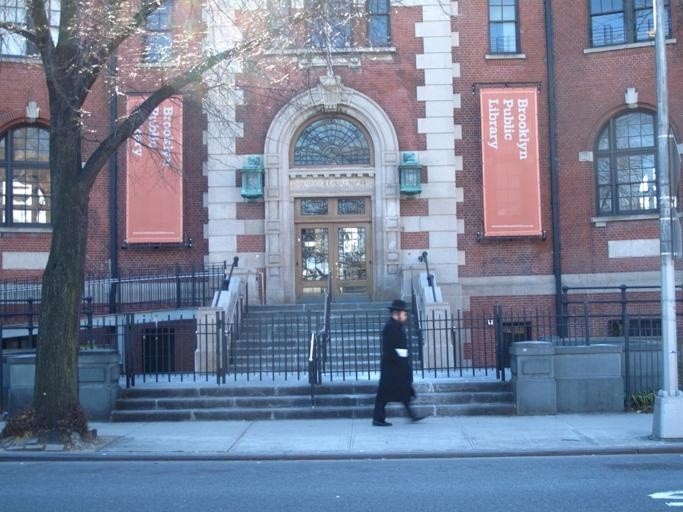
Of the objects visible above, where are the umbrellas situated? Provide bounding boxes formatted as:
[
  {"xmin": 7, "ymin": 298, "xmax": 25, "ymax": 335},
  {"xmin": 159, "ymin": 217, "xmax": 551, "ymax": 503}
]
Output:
[
  {"xmin": 413, "ymin": 415, "xmax": 424, "ymax": 420},
  {"xmin": 372, "ymin": 420, "xmax": 392, "ymax": 426}
]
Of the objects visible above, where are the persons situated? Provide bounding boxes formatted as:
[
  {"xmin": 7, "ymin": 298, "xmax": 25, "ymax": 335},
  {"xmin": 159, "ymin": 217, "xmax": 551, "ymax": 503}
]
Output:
[{"xmin": 372, "ymin": 299, "xmax": 433, "ymax": 426}]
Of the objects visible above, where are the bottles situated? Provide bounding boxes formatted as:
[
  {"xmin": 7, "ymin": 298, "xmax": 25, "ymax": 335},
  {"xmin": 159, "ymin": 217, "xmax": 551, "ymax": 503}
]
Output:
[{"xmin": 387, "ymin": 300, "xmax": 409, "ymax": 311}]
